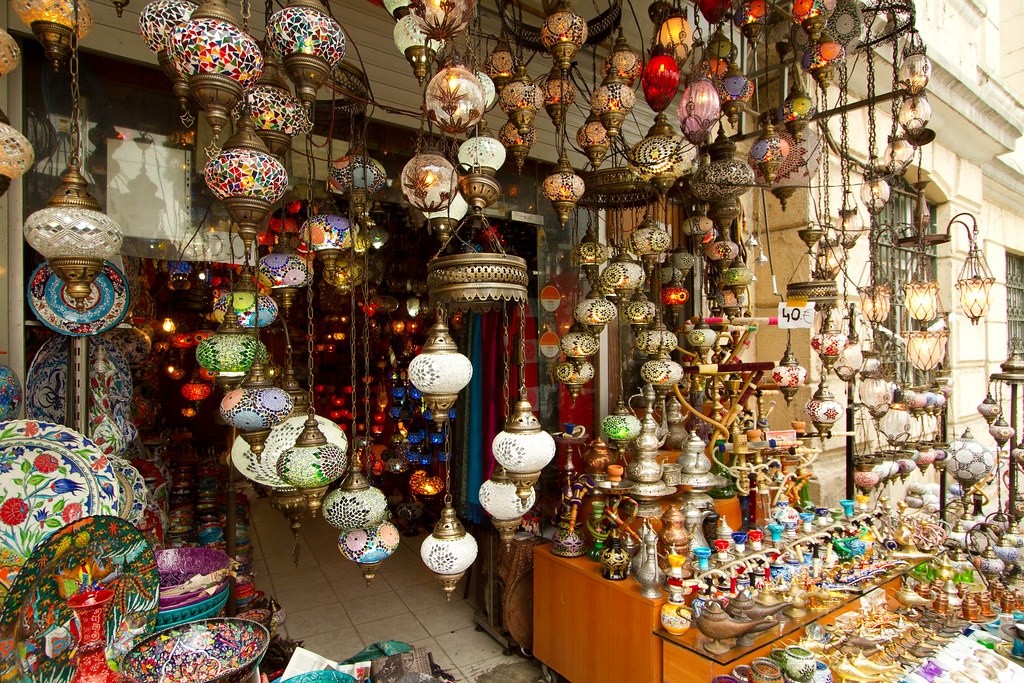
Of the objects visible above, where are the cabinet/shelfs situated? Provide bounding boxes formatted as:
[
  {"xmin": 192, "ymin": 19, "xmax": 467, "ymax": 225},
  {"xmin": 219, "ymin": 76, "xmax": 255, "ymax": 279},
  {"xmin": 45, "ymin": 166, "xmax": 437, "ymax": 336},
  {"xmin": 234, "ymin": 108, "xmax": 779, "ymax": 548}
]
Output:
[
  {"xmin": 652, "ymin": 544, "xmax": 972, "ymax": 683},
  {"xmin": 532, "ymin": 543, "xmax": 694, "ymax": 683}
]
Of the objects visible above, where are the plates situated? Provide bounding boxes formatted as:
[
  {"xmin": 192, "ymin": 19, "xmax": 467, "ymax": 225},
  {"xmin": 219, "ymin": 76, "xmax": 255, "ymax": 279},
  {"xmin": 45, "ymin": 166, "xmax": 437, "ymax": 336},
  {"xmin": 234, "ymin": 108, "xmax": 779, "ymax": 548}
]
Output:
[{"xmin": 0, "ymin": 261, "xmax": 266, "ymax": 683}]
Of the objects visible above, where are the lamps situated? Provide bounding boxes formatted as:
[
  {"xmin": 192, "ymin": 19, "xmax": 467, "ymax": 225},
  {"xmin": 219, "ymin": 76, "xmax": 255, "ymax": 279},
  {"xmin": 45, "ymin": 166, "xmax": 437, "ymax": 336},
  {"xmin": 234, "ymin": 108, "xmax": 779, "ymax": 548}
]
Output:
[{"xmin": 0, "ymin": 0, "xmax": 1024, "ymax": 604}]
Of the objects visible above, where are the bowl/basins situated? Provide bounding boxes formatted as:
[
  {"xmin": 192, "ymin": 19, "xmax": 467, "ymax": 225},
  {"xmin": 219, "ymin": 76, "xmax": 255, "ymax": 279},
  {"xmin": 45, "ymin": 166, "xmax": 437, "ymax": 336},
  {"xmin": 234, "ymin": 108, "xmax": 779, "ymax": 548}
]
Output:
[{"xmin": 169, "ymin": 466, "xmax": 271, "ymax": 627}]
